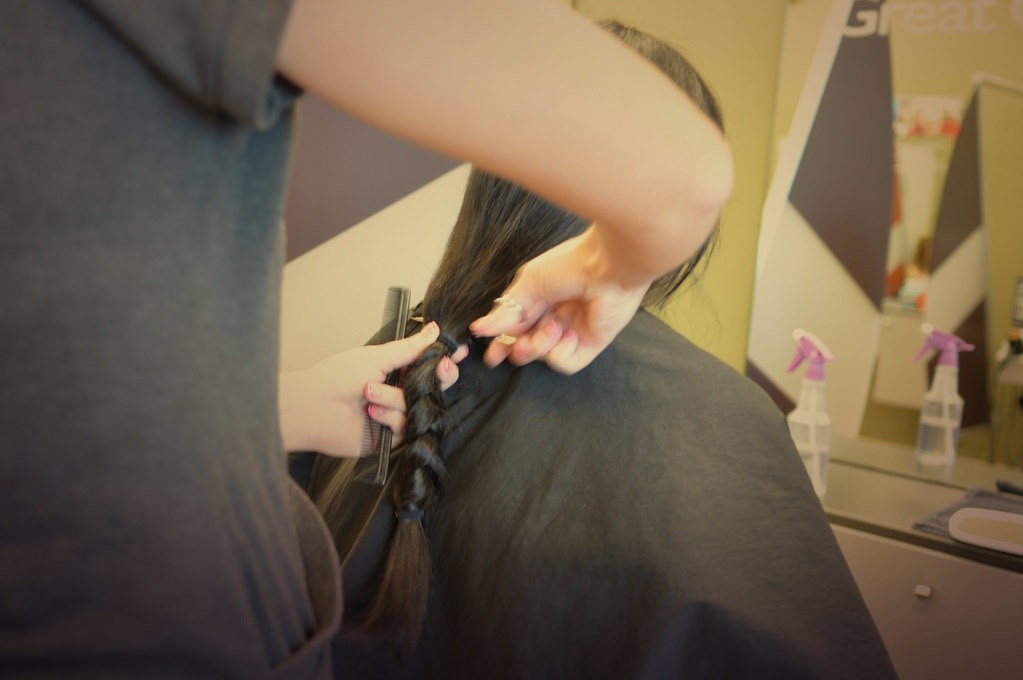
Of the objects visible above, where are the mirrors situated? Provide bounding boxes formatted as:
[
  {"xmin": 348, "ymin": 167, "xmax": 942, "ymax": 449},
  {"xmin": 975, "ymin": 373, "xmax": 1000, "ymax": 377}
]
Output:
[{"xmin": 741, "ymin": 0, "xmax": 1023, "ymax": 494}]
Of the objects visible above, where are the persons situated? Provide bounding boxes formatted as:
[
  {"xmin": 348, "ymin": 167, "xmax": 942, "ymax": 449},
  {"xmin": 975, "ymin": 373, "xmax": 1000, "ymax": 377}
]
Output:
[
  {"xmin": 1, "ymin": 0, "xmax": 735, "ymax": 679},
  {"xmin": 289, "ymin": 18, "xmax": 900, "ymax": 680}
]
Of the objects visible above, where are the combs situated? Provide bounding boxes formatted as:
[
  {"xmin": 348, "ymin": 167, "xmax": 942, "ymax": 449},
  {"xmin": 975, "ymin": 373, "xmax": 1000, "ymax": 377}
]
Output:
[{"xmin": 354, "ymin": 286, "xmax": 411, "ymax": 485}]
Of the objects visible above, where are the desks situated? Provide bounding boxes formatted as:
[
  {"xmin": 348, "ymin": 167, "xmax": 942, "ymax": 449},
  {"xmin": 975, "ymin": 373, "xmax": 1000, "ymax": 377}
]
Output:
[{"xmin": 821, "ymin": 459, "xmax": 1023, "ymax": 680}]
